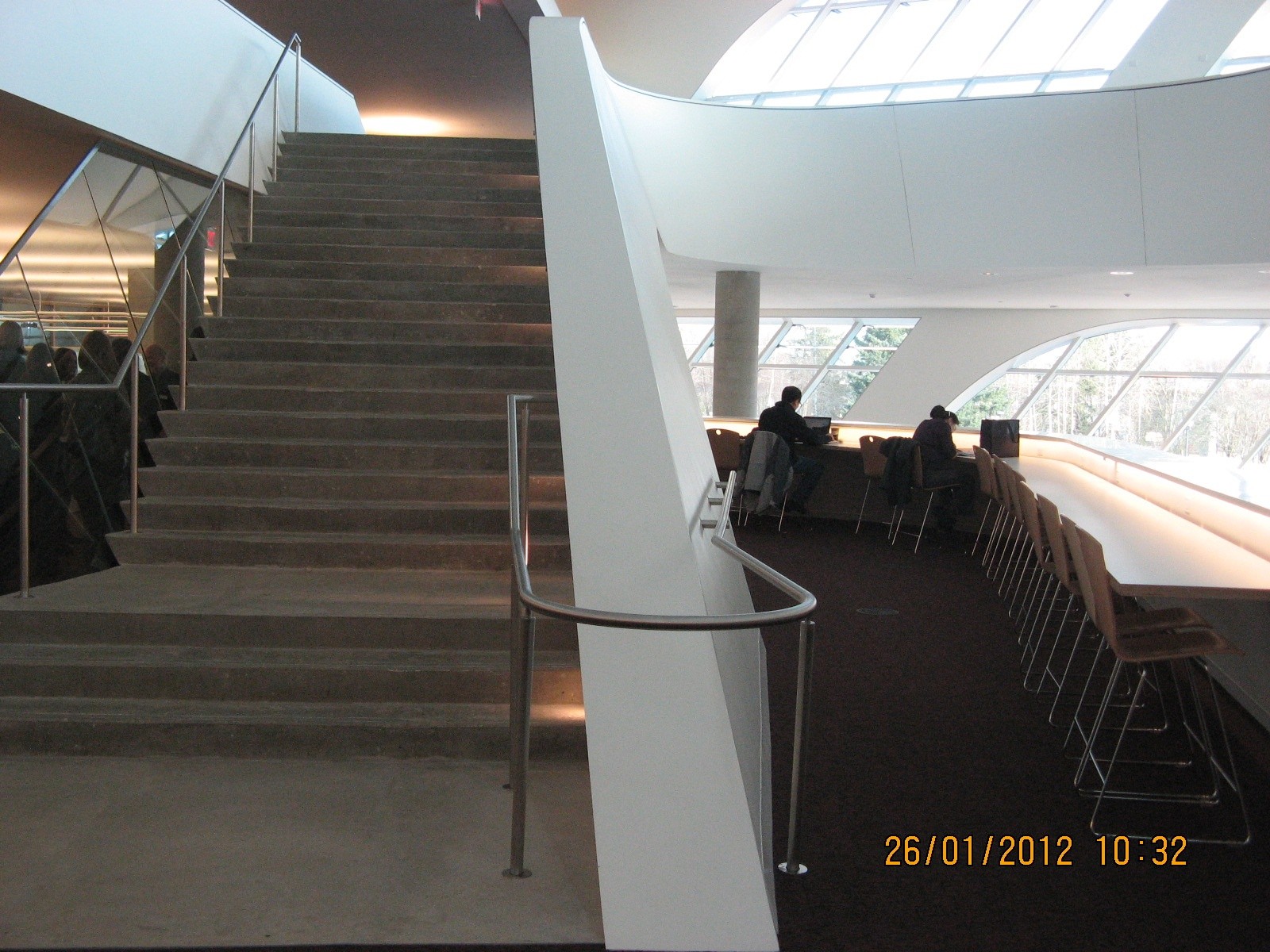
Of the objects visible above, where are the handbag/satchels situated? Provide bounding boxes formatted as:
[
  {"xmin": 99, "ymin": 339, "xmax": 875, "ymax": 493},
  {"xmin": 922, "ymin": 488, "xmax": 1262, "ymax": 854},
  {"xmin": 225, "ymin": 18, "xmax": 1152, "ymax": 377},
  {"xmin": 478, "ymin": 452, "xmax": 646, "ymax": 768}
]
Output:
[{"xmin": 980, "ymin": 419, "xmax": 1020, "ymax": 457}]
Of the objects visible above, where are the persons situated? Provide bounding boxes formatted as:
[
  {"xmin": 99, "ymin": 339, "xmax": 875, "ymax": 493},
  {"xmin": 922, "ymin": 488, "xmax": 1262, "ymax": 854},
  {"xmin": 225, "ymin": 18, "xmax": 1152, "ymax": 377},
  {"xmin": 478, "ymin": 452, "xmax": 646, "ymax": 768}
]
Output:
[
  {"xmin": 913, "ymin": 406, "xmax": 978, "ymax": 534},
  {"xmin": 758, "ymin": 386, "xmax": 825, "ymax": 514},
  {"xmin": 0, "ymin": 319, "xmax": 183, "ymax": 570}
]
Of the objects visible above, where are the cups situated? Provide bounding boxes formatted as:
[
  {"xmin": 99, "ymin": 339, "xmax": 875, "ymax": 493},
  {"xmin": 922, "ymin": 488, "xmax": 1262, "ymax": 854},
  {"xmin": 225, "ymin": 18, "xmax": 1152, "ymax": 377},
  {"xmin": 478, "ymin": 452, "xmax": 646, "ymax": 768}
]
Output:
[{"xmin": 831, "ymin": 428, "xmax": 839, "ymax": 440}]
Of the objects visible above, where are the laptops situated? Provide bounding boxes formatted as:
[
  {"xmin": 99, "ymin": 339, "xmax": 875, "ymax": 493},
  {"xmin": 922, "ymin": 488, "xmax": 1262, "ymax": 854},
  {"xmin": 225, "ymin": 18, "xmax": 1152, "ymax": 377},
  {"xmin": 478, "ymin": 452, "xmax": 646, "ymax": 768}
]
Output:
[{"xmin": 794, "ymin": 416, "xmax": 832, "ymax": 442}]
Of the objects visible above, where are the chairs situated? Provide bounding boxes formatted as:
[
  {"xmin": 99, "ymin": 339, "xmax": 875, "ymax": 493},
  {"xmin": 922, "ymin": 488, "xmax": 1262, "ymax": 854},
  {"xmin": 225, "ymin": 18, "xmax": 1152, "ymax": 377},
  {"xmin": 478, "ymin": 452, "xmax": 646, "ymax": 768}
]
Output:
[{"xmin": 707, "ymin": 429, "xmax": 1253, "ymax": 846}]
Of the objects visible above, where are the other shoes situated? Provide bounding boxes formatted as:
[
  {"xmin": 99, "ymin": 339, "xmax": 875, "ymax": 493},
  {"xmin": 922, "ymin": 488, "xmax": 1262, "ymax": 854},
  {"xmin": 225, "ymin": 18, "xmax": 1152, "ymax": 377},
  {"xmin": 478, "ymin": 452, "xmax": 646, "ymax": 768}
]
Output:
[{"xmin": 780, "ymin": 500, "xmax": 807, "ymax": 512}]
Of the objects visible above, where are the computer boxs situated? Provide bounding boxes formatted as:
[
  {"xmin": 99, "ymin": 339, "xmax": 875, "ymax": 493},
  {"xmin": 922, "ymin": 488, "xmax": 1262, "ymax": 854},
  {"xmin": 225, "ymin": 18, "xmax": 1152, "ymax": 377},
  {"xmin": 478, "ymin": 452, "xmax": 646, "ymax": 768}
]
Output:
[{"xmin": 980, "ymin": 419, "xmax": 1020, "ymax": 459}]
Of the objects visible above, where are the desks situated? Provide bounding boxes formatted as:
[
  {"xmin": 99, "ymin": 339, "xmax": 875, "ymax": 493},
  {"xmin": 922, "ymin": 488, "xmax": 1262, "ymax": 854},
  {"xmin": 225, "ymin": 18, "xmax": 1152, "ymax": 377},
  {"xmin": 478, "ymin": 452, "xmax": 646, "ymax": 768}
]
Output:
[{"xmin": 740, "ymin": 429, "xmax": 1270, "ymax": 600}]
